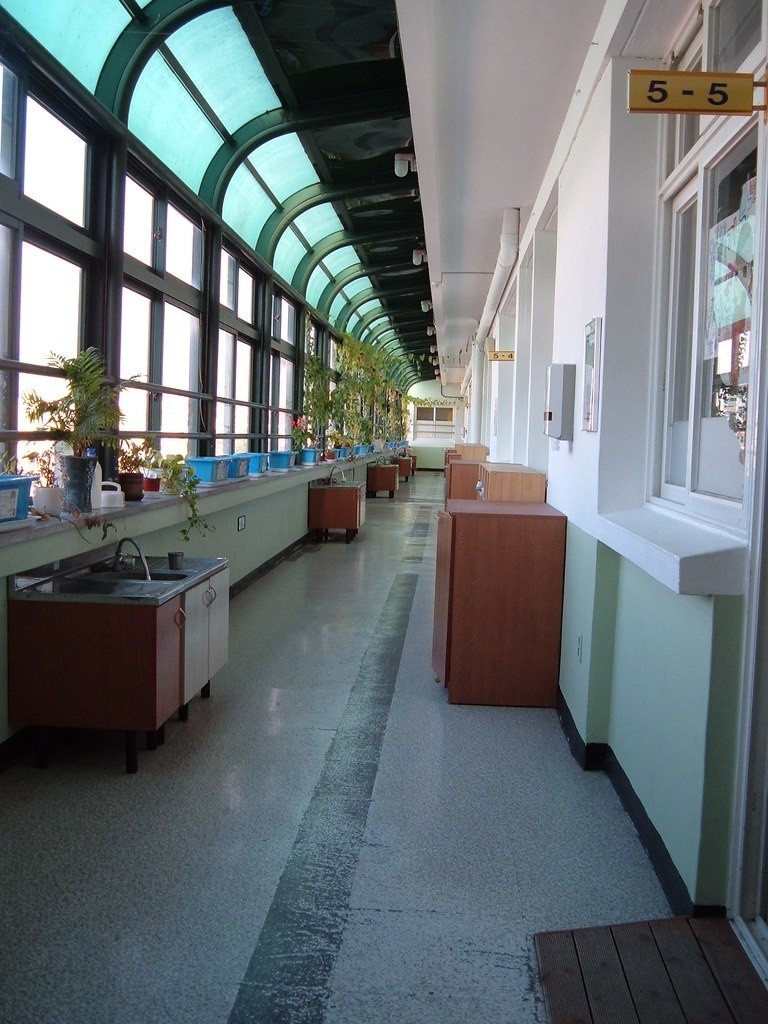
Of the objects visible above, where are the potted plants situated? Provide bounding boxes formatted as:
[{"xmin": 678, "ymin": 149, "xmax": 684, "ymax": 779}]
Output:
[
  {"xmin": 325, "ymin": 332, "xmax": 409, "ymax": 459},
  {"xmin": 22, "ymin": 346, "xmax": 140, "ymax": 512},
  {"xmin": 154, "ymin": 454, "xmax": 217, "ymax": 542},
  {"xmin": 22, "ymin": 450, "xmax": 117, "ymax": 543},
  {"xmin": 118, "ymin": 434, "xmax": 156, "ymax": 502},
  {"xmin": 140, "ymin": 459, "xmax": 164, "ymax": 499}
]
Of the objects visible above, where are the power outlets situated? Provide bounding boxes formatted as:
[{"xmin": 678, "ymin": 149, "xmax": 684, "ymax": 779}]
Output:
[
  {"xmin": 576, "ymin": 632, "xmax": 583, "ymax": 663},
  {"xmin": 238, "ymin": 515, "xmax": 246, "ymax": 532}
]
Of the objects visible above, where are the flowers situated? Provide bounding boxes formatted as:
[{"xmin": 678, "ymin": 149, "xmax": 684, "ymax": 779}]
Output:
[{"xmin": 290, "ymin": 416, "xmax": 317, "ymax": 450}]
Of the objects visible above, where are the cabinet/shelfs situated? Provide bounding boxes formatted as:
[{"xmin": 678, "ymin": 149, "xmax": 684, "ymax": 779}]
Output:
[
  {"xmin": 368, "ymin": 456, "xmax": 416, "ymax": 491},
  {"xmin": 432, "ymin": 499, "xmax": 567, "ymax": 708},
  {"xmin": 444, "ymin": 444, "xmax": 487, "ymax": 478},
  {"xmin": 180, "ymin": 568, "xmax": 229, "ymax": 706},
  {"xmin": 8, "ymin": 593, "xmax": 187, "ymax": 732},
  {"xmin": 308, "ymin": 485, "xmax": 366, "ymax": 528},
  {"xmin": 475, "ymin": 463, "xmax": 546, "ymax": 502},
  {"xmin": 445, "ymin": 460, "xmax": 490, "ymax": 513}
]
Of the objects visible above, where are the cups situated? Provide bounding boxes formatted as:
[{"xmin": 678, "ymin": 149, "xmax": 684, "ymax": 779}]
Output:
[{"xmin": 167, "ymin": 551, "xmax": 184, "ymax": 571}]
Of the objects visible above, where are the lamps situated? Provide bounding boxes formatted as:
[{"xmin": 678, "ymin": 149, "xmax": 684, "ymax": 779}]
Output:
[
  {"xmin": 430, "ymin": 346, "xmax": 438, "ymax": 353},
  {"xmin": 395, "ymin": 154, "xmax": 417, "ymax": 177},
  {"xmin": 435, "ymin": 369, "xmax": 440, "ymax": 375},
  {"xmin": 413, "ymin": 250, "xmax": 428, "ymax": 265},
  {"xmin": 433, "ymin": 359, "xmax": 439, "ymax": 366},
  {"xmin": 421, "ymin": 301, "xmax": 433, "ymax": 312},
  {"xmin": 427, "ymin": 327, "xmax": 436, "ymax": 336}
]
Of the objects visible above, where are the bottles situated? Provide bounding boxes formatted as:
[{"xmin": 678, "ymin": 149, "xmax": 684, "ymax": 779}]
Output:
[
  {"xmin": 85, "ymin": 447, "xmax": 103, "ymax": 509},
  {"xmin": 475, "ymin": 480, "xmax": 485, "ymax": 506}
]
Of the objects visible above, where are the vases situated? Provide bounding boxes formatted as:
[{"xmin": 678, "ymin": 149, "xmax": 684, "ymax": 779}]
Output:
[
  {"xmin": 296, "ymin": 449, "xmax": 323, "ymax": 466},
  {"xmin": 186, "ymin": 456, "xmax": 233, "ymax": 488},
  {"xmin": 233, "ymin": 452, "xmax": 271, "ymax": 478},
  {"xmin": 0, "ymin": 475, "xmax": 42, "ymax": 533},
  {"xmin": 215, "ymin": 455, "xmax": 253, "ymax": 483},
  {"xmin": 267, "ymin": 451, "xmax": 299, "ymax": 472}
]
